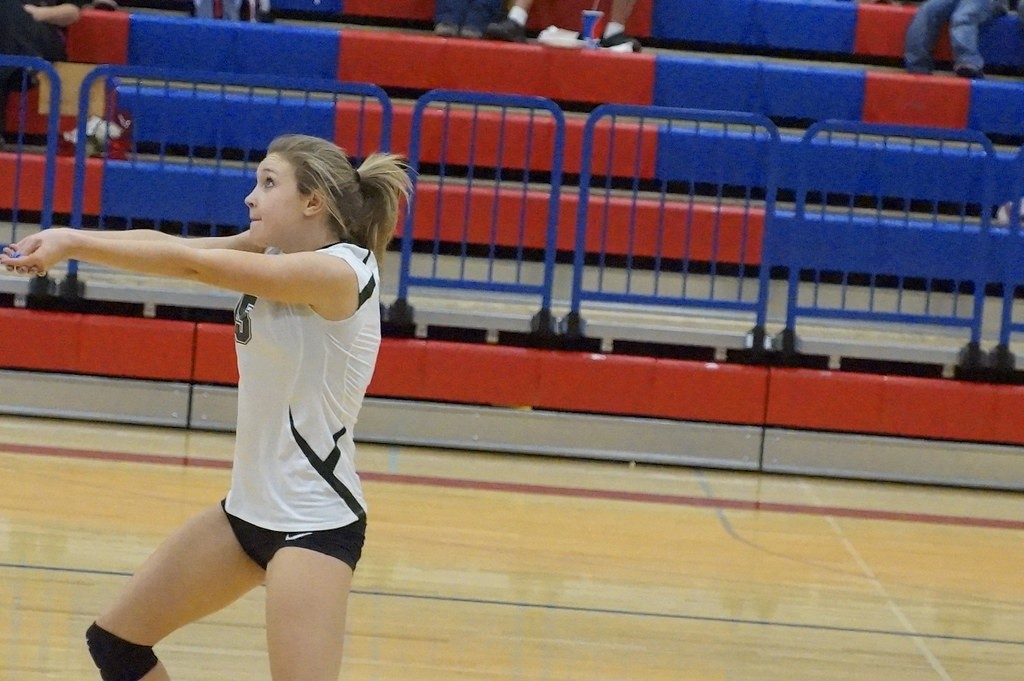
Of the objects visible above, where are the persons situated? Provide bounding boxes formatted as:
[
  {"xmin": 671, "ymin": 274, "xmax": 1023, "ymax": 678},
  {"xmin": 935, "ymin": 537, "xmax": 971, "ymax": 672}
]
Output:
[
  {"xmin": 0, "ymin": 0, "xmax": 81, "ymax": 132},
  {"xmin": 431, "ymin": 0, "xmax": 643, "ymax": 54},
  {"xmin": 903, "ymin": 0, "xmax": 1009, "ymax": 79},
  {"xmin": 0, "ymin": 131, "xmax": 417, "ymax": 681}
]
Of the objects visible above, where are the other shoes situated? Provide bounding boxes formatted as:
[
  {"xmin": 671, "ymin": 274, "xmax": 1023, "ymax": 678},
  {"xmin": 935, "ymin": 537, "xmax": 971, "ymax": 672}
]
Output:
[
  {"xmin": 487, "ymin": 19, "xmax": 528, "ymax": 43},
  {"xmin": 596, "ymin": 32, "xmax": 642, "ymax": 52},
  {"xmin": 955, "ymin": 63, "xmax": 984, "ymax": 80},
  {"xmin": 461, "ymin": 25, "xmax": 482, "ymax": 38},
  {"xmin": 433, "ymin": 21, "xmax": 459, "ymax": 37}
]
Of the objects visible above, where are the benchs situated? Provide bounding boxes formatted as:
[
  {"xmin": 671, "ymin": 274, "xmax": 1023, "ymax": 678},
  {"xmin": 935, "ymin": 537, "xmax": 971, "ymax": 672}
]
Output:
[{"xmin": 1, "ymin": 0, "xmax": 1024, "ymax": 286}]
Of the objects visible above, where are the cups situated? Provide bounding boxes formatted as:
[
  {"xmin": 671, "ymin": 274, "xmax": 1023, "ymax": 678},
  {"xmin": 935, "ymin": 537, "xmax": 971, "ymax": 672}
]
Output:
[{"xmin": 583, "ymin": 9, "xmax": 605, "ymax": 46}]
